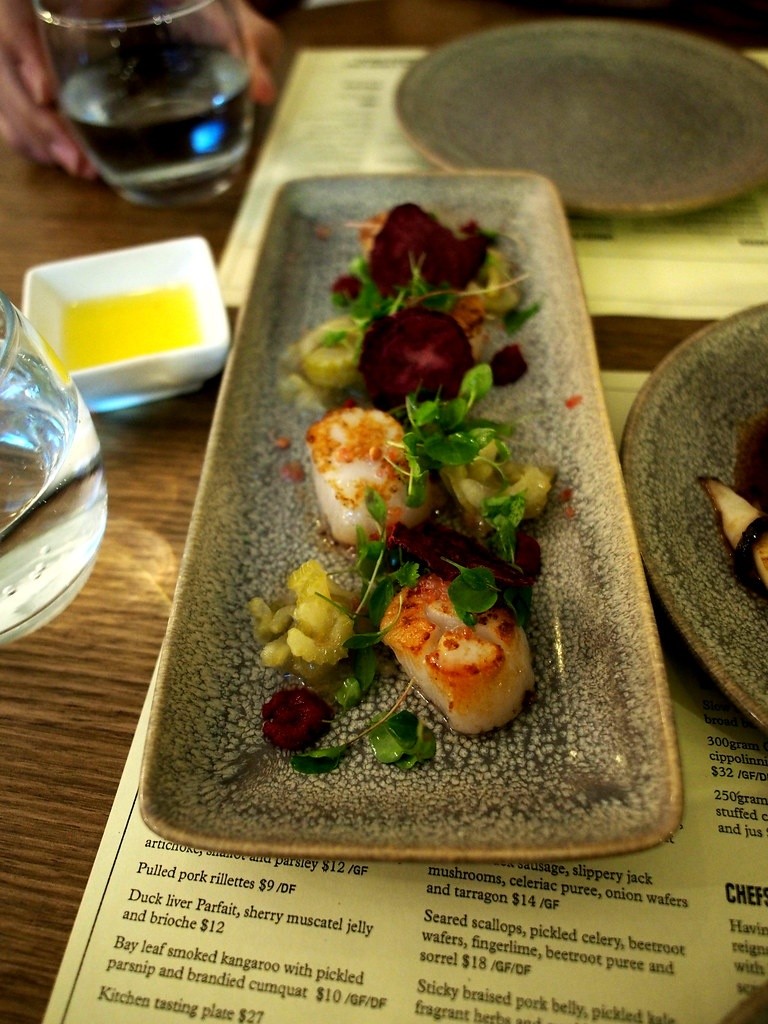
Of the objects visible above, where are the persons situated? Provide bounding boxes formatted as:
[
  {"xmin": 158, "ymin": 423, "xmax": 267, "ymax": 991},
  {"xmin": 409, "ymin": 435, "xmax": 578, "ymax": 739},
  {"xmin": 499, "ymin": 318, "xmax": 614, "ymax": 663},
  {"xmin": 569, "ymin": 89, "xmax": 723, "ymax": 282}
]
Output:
[{"xmin": 1, "ymin": 0, "xmax": 307, "ymax": 181}]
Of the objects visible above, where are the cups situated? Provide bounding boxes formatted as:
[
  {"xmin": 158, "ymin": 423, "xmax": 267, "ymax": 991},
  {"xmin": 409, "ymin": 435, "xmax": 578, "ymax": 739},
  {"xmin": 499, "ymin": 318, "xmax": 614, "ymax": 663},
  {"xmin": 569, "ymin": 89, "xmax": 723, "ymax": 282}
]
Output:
[
  {"xmin": 0, "ymin": 292, "xmax": 108, "ymax": 645},
  {"xmin": 35, "ymin": 0, "xmax": 254, "ymax": 209}
]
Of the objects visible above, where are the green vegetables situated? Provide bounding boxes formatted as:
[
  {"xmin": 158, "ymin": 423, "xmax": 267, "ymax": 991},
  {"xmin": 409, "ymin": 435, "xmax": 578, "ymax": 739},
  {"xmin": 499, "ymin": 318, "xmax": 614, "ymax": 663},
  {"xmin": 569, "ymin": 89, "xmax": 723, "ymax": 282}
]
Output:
[{"xmin": 291, "ymin": 259, "xmax": 540, "ymax": 775}]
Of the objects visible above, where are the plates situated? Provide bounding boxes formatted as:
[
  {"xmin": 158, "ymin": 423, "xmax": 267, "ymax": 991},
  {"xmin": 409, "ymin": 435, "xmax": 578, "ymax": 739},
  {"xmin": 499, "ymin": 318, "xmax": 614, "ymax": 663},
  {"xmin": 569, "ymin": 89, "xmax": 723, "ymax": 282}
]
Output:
[
  {"xmin": 139, "ymin": 168, "xmax": 686, "ymax": 863},
  {"xmin": 620, "ymin": 300, "xmax": 768, "ymax": 734},
  {"xmin": 392, "ymin": 20, "xmax": 768, "ymax": 219},
  {"xmin": 21, "ymin": 234, "xmax": 231, "ymax": 413}
]
